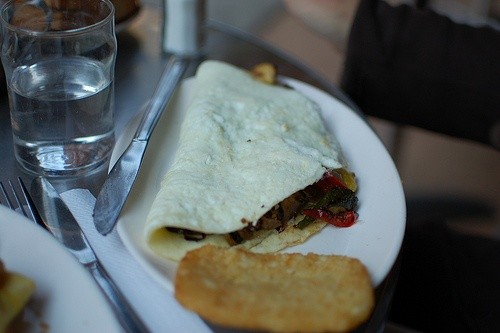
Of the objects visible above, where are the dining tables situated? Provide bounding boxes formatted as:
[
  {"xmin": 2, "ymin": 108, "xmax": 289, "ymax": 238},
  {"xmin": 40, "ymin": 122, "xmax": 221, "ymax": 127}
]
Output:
[{"xmin": 0, "ymin": 0, "xmax": 390, "ymax": 333}]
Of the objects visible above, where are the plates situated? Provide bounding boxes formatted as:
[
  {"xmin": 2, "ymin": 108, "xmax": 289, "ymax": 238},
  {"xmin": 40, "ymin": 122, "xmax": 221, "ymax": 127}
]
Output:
[
  {"xmin": 0, "ymin": 204, "xmax": 127, "ymax": 333},
  {"xmin": 114, "ymin": 70, "xmax": 406, "ymax": 294},
  {"xmin": 10, "ymin": 0, "xmax": 147, "ymax": 38}
]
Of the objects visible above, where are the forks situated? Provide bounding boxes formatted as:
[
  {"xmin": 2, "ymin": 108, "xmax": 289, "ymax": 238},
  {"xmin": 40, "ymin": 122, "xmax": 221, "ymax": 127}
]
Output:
[{"xmin": 0, "ymin": 177, "xmax": 47, "ymax": 229}]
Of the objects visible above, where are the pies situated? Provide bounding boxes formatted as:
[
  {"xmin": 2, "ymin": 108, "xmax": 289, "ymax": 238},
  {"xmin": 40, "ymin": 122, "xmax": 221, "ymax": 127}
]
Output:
[{"xmin": 143, "ymin": 59, "xmax": 359, "ymax": 260}]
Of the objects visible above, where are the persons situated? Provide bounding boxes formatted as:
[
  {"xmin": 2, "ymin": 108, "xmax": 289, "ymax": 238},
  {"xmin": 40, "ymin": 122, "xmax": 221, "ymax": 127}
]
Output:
[{"xmin": 270, "ymin": 0, "xmax": 500, "ymax": 150}]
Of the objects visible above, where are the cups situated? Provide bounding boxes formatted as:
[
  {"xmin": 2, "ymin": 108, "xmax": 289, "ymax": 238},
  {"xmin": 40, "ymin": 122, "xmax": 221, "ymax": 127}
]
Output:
[{"xmin": 0, "ymin": 0, "xmax": 118, "ymax": 179}]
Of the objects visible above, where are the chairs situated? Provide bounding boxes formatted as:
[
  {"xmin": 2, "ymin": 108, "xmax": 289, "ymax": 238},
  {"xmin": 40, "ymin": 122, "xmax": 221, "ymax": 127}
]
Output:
[{"xmin": 327, "ymin": 1, "xmax": 500, "ymax": 333}]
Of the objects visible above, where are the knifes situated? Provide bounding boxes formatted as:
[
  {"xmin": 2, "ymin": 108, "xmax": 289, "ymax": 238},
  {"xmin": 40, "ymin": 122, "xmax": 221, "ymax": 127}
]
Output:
[
  {"xmin": 24, "ymin": 176, "xmax": 151, "ymax": 333},
  {"xmin": 93, "ymin": 56, "xmax": 190, "ymax": 236}
]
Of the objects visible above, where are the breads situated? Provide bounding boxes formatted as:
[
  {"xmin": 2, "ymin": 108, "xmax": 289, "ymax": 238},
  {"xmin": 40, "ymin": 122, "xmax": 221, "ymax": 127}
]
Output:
[
  {"xmin": 0, "ymin": 270, "xmax": 36, "ymax": 333},
  {"xmin": 174, "ymin": 244, "xmax": 374, "ymax": 333}
]
